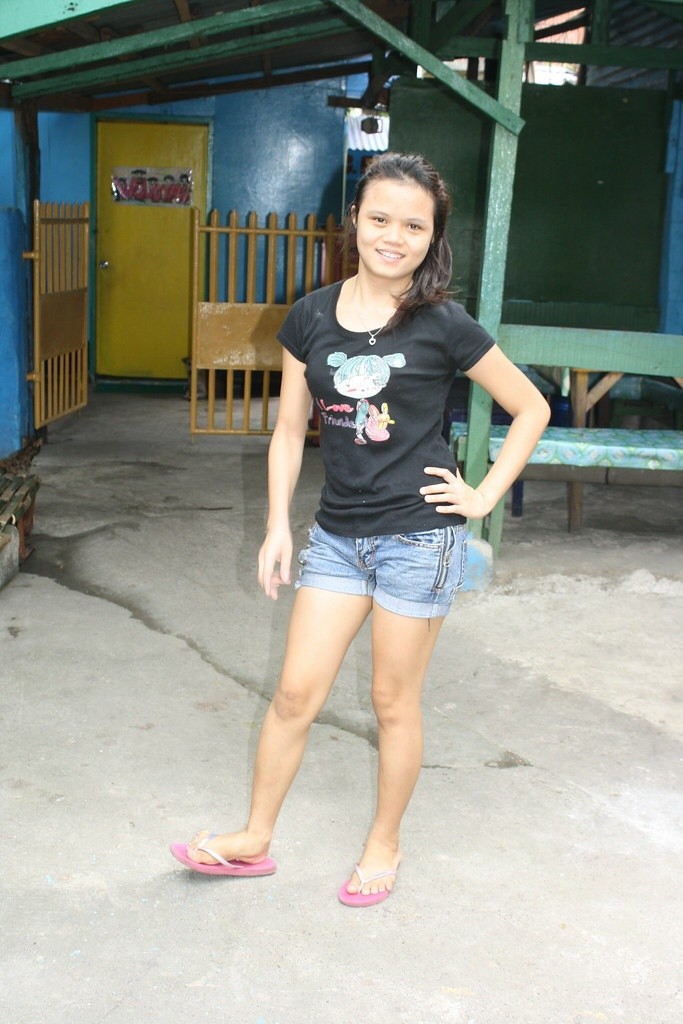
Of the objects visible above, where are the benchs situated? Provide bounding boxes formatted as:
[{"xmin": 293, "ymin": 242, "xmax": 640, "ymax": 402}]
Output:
[{"xmin": 448, "ymin": 323, "xmax": 683, "ymax": 554}]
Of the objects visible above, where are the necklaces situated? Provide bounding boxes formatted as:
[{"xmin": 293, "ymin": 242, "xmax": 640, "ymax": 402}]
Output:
[{"xmin": 355, "ymin": 295, "xmax": 398, "ymax": 345}]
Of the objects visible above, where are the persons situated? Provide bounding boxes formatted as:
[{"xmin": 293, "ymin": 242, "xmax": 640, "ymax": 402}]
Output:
[{"xmin": 169, "ymin": 153, "xmax": 551, "ymax": 907}]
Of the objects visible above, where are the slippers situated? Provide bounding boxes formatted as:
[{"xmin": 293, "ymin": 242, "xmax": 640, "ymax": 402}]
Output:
[
  {"xmin": 171, "ymin": 833, "xmax": 275, "ymax": 877},
  {"xmin": 338, "ymin": 850, "xmax": 401, "ymax": 907}
]
